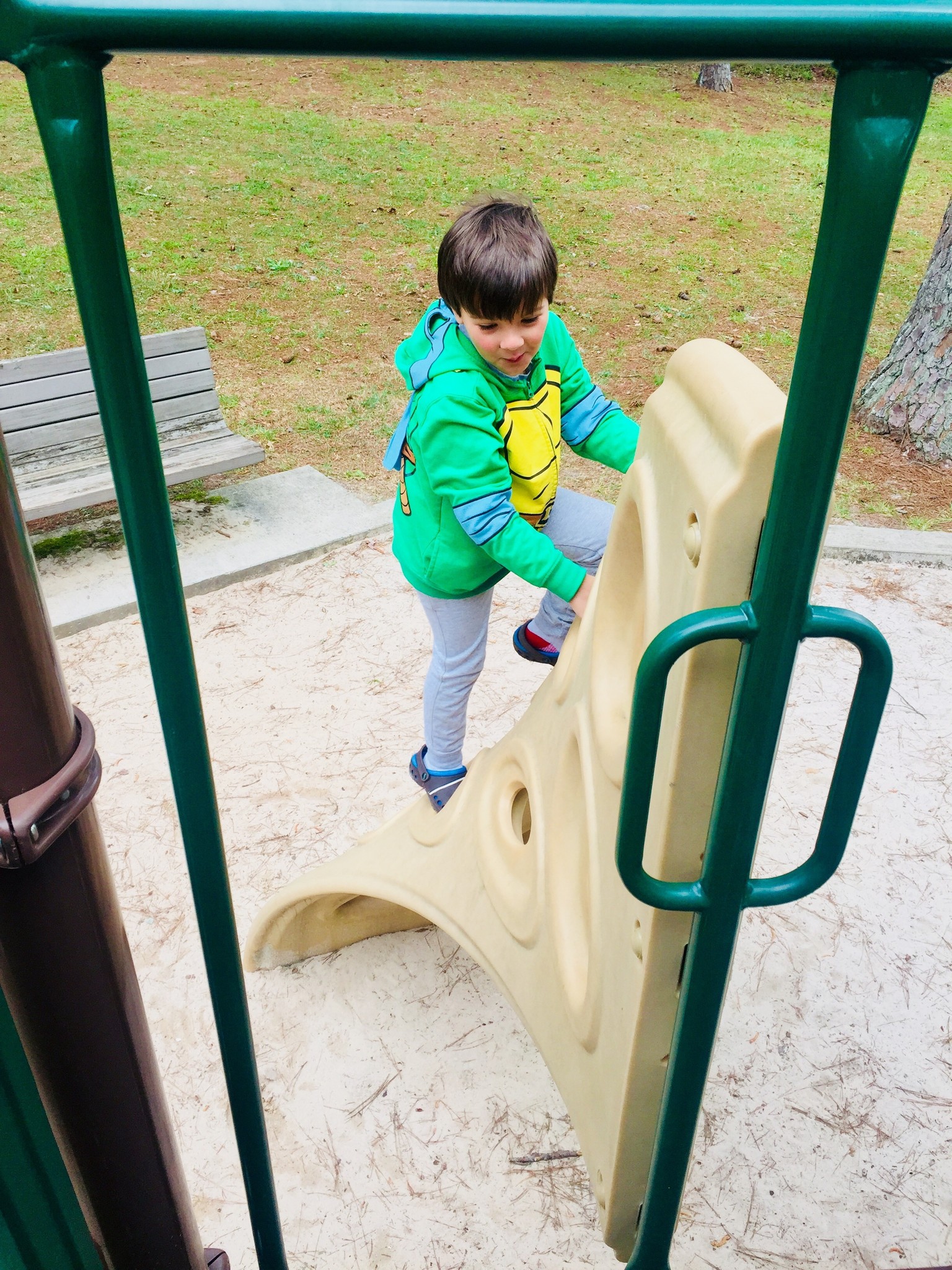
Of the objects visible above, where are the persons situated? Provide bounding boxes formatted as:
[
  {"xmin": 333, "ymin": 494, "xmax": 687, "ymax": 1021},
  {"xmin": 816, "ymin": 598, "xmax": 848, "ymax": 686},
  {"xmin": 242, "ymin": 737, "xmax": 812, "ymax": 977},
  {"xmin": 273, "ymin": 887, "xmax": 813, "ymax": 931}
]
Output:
[{"xmin": 383, "ymin": 199, "xmax": 641, "ymax": 812}]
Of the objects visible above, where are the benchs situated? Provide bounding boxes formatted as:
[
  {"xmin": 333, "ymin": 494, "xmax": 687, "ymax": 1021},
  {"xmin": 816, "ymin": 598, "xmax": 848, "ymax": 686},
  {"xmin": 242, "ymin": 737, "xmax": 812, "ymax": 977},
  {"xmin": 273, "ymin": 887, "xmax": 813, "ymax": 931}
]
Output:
[{"xmin": 0, "ymin": 326, "xmax": 267, "ymax": 524}]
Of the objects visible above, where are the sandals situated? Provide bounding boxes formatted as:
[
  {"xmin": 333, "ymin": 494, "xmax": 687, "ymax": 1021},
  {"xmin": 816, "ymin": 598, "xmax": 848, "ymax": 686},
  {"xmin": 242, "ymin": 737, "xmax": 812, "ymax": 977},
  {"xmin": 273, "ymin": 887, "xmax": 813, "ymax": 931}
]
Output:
[
  {"xmin": 513, "ymin": 619, "xmax": 560, "ymax": 667},
  {"xmin": 409, "ymin": 745, "xmax": 468, "ymax": 813}
]
